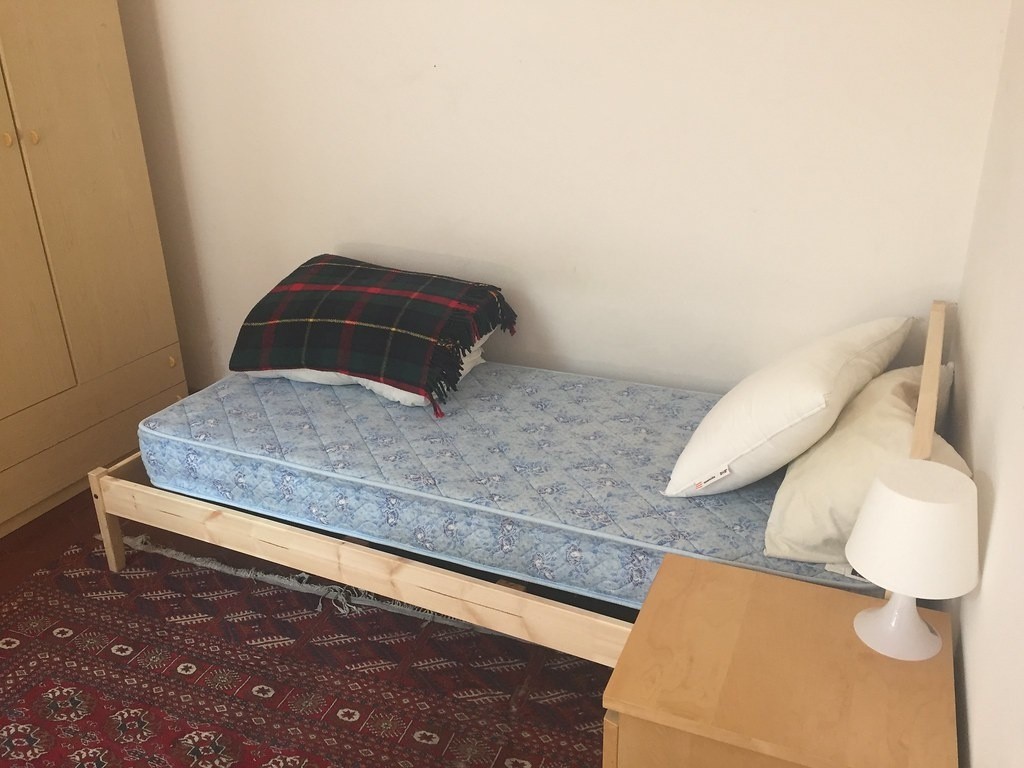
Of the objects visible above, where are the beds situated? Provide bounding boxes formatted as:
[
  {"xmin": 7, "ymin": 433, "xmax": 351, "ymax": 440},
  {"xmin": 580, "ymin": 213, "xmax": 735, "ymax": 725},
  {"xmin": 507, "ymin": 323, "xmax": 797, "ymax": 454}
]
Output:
[{"xmin": 85, "ymin": 297, "xmax": 953, "ymax": 672}]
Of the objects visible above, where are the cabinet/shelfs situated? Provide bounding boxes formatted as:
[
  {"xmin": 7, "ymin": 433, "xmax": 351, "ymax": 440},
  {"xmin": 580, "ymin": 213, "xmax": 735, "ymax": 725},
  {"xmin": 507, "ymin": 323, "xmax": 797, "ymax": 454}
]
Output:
[{"xmin": 0, "ymin": 0, "xmax": 190, "ymax": 542}]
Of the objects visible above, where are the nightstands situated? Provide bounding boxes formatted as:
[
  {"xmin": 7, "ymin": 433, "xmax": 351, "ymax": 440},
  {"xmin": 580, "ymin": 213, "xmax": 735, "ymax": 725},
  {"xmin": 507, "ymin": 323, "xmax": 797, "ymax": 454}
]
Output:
[{"xmin": 602, "ymin": 554, "xmax": 961, "ymax": 768}]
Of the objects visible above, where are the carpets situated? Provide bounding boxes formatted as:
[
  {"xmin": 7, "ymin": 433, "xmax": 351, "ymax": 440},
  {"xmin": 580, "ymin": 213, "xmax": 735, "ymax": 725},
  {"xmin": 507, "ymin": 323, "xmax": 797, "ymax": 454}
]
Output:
[{"xmin": 0, "ymin": 533, "xmax": 613, "ymax": 768}]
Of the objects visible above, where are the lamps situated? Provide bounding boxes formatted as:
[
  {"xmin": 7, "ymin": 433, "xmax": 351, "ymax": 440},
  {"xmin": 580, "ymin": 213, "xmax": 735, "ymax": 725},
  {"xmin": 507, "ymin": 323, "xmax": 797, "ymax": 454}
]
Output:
[{"xmin": 842, "ymin": 459, "xmax": 983, "ymax": 663}]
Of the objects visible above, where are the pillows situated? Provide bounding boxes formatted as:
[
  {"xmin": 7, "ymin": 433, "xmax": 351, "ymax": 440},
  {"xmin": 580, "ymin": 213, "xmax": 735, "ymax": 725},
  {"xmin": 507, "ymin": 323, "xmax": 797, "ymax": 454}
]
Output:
[
  {"xmin": 661, "ymin": 315, "xmax": 913, "ymax": 497},
  {"xmin": 761, "ymin": 362, "xmax": 972, "ymax": 563}
]
